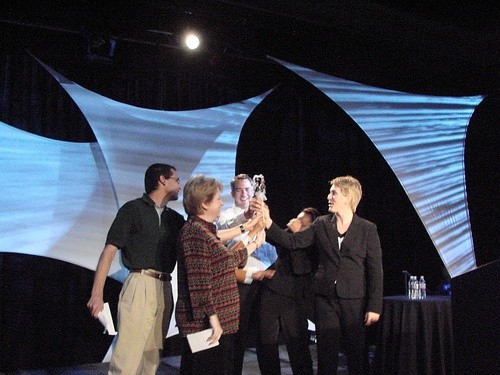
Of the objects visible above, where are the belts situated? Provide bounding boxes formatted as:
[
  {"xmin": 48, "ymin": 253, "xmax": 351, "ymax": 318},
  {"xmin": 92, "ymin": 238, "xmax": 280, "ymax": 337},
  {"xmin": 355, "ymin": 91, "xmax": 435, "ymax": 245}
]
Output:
[{"xmin": 129, "ymin": 268, "xmax": 172, "ymax": 282}]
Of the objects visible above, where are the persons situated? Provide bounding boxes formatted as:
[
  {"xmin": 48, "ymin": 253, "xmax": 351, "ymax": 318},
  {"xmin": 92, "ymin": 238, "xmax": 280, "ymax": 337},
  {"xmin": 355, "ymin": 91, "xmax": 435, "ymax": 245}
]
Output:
[
  {"xmin": 226, "ymin": 218, "xmax": 267, "ymax": 285},
  {"xmin": 87, "ymin": 162, "xmax": 264, "ymax": 375},
  {"xmin": 175, "ymin": 174, "xmax": 267, "ymax": 375},
  {"xmin": 254, "ymin": 208, "xmax": 319, "ymax": 375},
  {"xmin": 217, "ymin": 173, "xmax": 263, "ymax": 374},
  {"xmin": 260, "ymin": 175, "xmax": 383, "ymax": 375}
]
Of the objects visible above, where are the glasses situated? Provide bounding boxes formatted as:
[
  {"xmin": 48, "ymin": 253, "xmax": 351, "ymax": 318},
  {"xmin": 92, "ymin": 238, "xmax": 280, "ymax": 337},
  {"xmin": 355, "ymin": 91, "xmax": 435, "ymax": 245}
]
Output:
[{"xmin": 169, "ymin": 177, "xmax": 180, "ymax": 184}]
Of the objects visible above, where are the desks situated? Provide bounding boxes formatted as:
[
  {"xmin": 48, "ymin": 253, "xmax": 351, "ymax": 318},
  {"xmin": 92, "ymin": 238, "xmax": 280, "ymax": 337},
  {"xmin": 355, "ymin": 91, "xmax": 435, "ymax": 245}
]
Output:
[{"xmin": 379, "ymin": 297, "xmax": 453, "ymax": 375}]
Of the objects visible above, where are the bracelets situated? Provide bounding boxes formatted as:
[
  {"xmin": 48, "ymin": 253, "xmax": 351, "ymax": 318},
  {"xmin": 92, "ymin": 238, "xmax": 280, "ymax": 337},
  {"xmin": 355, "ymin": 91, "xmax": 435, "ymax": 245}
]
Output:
[
  {"xmin": 243, "ymin": 269, "xmax": 253, "ymax": 285},
  {"xmin": 238, "ymin": 224, "xmax": 246, "ymax": 234},
  {"xmin": 252, "ymin": 241, "xmax": 258, "ymax": 249},
  {"xmin": 241, "ymin": 236, "xmax": 252, "ymax": 246}
]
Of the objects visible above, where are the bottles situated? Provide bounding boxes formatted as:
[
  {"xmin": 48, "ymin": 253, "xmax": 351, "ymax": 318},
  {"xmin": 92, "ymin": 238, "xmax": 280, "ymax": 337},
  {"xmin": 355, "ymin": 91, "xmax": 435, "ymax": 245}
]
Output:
[{"xmin": 407, "ymin": 276, "xmax": 426, "ymax": 300}]
